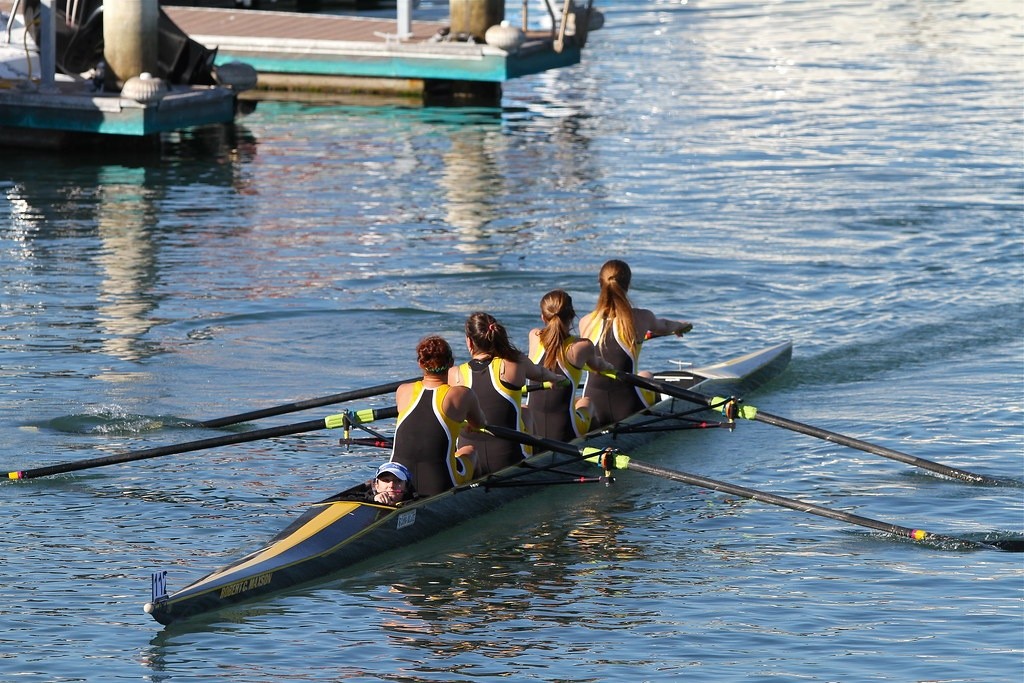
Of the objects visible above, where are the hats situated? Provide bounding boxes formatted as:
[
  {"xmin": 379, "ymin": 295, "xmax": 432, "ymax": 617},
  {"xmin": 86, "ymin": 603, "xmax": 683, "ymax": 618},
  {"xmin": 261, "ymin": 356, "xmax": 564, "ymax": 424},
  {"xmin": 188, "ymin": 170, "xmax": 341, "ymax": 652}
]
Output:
[{"xmin": 373, "ymin": 462, "xmax": 409, "ymax": 482}]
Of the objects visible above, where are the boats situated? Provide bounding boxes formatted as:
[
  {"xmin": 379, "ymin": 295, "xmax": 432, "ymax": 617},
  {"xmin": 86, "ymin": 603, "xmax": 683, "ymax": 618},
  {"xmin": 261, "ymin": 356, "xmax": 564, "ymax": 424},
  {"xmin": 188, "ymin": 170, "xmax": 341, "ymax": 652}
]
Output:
[{"xmin": 144, "ymin": 341, "xmax": 793, "ymax": 626}]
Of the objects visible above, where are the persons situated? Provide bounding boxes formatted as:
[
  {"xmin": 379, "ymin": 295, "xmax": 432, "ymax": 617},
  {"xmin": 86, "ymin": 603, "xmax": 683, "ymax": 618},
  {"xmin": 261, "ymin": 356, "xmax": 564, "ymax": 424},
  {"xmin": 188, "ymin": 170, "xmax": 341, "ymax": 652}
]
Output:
[
  {"xmin": 521, "ymin": 290, "xmax": 601, "ymax": 455},
  {"xmin": 449, "ymin": 311, "xmax": 565, "ymax": 476},
  {"xmin": 390, "ymin": 336, "xmax": 488, "ymax": 498},
  {"xmin": 329, "ymin": 462, "xmax": 421, "ymax": 507},
  {"xmin": 579, "ymin": 259, "xmax": 693, "ymax": 424}
]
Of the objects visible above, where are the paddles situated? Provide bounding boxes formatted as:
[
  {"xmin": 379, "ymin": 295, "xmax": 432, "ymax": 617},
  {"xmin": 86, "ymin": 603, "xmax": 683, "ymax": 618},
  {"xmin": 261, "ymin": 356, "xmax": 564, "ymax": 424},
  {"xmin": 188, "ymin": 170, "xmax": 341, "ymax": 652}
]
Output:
[
  {"xmin": 0, "ymin": 404, "xmax": 401, "ymax": 484},
  {"xmin": 578, "ymin": 358, "xmax": 1024, "ymax": 489},
  {"xmin": 175, "ymin": 369, "xmax": 425, "ymax": 431},
  {"xmin": 456, "ymin": 415, "xmax": 1024, "ymax": 570}
]
